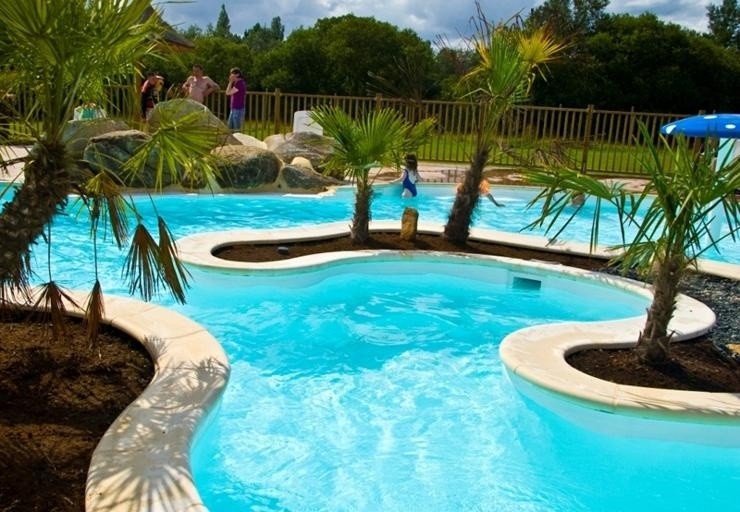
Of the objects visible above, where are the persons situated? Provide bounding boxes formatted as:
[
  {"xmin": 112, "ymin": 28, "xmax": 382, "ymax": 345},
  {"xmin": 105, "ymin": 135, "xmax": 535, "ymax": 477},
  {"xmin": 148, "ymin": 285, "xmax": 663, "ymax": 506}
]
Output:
[
  {"xmin": 183, "ymin": 65, "xmax": 222, "ymax": 104},
  {"xmin": 142, "ymin": 71, "xmax": 165, "ymax": 122},
  {"xmin": 480, "ymin": 180, "xmax": 506, "ymax": 208},
  {"xmin": 390, "ymin": 154, "xmax": 423, "ymax": 196},
  {"xmin": 226, "ymin": 67, "xmax": 247, "ymax": 130},
  {"xmin": 73, "ymin": 100, "xmax": 108, "ymax": 121}
]
